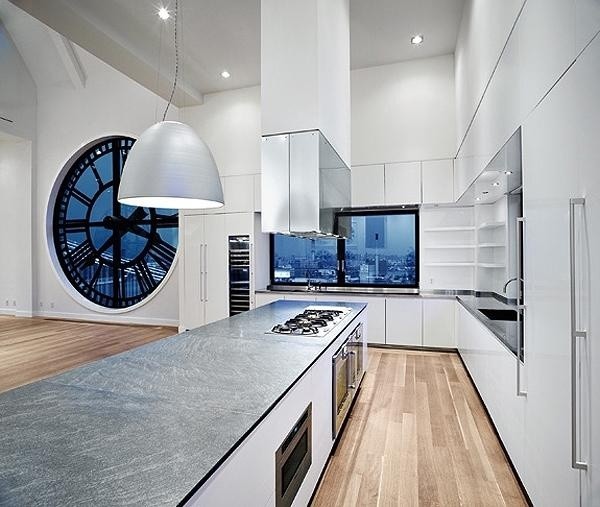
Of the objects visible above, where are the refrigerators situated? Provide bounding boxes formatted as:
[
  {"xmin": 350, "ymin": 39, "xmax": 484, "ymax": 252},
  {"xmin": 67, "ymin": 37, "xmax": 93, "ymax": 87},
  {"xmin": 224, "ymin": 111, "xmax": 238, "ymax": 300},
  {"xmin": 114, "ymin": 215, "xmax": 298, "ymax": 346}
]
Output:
[{"xmin": 182, "ymin": 211, "xmax": 271, "ymax": 339}]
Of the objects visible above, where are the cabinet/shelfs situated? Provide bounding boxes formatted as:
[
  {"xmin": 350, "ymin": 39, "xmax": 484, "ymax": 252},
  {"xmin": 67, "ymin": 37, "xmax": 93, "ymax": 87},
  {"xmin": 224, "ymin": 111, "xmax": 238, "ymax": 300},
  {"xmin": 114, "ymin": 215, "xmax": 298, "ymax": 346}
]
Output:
[
  {"xmin": 476, "ymin": 221, "xmax": 506, "ymax": 268},
  {"xmin": 521, "ymin": 34, "xmax": 600, "ymax": 506},
  {"xmin": 224, "ymin": 174, "xmax": 261, "ymax": 214},
  {"xmin": 255, "ymin": 293, "xmax": 316, "ymax": 311},
  {"xmin": 184, "ymin": 176, "xmax": 224, "ymax": 215},
  {"xmin": 424, "ymin": 226, "xmax": 475, "ymax": 267},
  {"xmin": 421, "ymin": 158, "xmax": 454, "ymax": 204},
  {"xmin": 351, "ymin": 160, "xmax": 421, "ymax": 206},
  {"xmin": 385, "ymin": 296, "xmax": 458, "ymax": 348},
  {"xmin": 457, "ymin": 296, "xmax": 523, "ymax": 493},
  {"xmin": 316, "ymin": 296, "xmax": 385, "ymax": 347}
]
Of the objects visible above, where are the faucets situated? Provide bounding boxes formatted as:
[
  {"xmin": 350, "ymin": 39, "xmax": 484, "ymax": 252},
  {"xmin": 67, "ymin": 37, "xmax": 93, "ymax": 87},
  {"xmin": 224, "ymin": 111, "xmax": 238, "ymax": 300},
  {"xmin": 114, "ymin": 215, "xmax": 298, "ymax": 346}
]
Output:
[{"xmin": 503, "ymin": 277, "xmax": 524, "ymax": 294}]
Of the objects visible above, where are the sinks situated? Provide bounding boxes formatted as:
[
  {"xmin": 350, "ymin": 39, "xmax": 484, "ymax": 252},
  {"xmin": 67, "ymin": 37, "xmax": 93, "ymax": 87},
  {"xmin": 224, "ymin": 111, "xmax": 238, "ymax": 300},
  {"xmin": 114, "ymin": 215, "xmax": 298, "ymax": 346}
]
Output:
[{"xmin": 476, "ymin": 307, "xmax": 523, "ymax": 322}]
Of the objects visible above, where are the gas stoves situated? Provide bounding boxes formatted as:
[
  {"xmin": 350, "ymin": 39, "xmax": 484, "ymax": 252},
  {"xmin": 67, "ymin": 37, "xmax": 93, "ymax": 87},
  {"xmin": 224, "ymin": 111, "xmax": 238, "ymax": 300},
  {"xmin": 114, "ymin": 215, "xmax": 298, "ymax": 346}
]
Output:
[{"xmin": 264, "ymin": 304, "xmax": 353, "ymax": 339}]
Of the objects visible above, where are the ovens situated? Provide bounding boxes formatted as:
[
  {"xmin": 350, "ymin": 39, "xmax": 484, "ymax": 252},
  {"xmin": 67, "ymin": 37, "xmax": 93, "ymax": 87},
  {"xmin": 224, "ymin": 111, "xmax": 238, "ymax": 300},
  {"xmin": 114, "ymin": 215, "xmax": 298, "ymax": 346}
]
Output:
[
  {"xmin": 331, "ymin": 322, "xmax": 364, "ymax": 442},
  {"xmin": 274, "ymin": 401, "xmax": 313, "ymax": 507}
]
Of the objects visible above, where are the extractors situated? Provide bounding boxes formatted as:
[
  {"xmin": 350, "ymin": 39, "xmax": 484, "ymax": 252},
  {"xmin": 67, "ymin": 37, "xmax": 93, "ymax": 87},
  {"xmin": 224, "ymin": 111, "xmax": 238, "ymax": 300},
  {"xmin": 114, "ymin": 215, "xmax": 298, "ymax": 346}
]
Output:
[{"xmin": 259, "ymin": 128, "xmax": 353, "ymax": 243}]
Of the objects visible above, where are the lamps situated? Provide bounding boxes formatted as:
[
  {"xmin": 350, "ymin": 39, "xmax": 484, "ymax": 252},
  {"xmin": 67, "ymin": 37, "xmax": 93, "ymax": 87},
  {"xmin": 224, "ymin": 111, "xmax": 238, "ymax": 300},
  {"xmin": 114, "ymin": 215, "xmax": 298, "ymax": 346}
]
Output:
[{"xmin": 114, "ymin": 0, "xmax": 227, "ymax": 210}]
